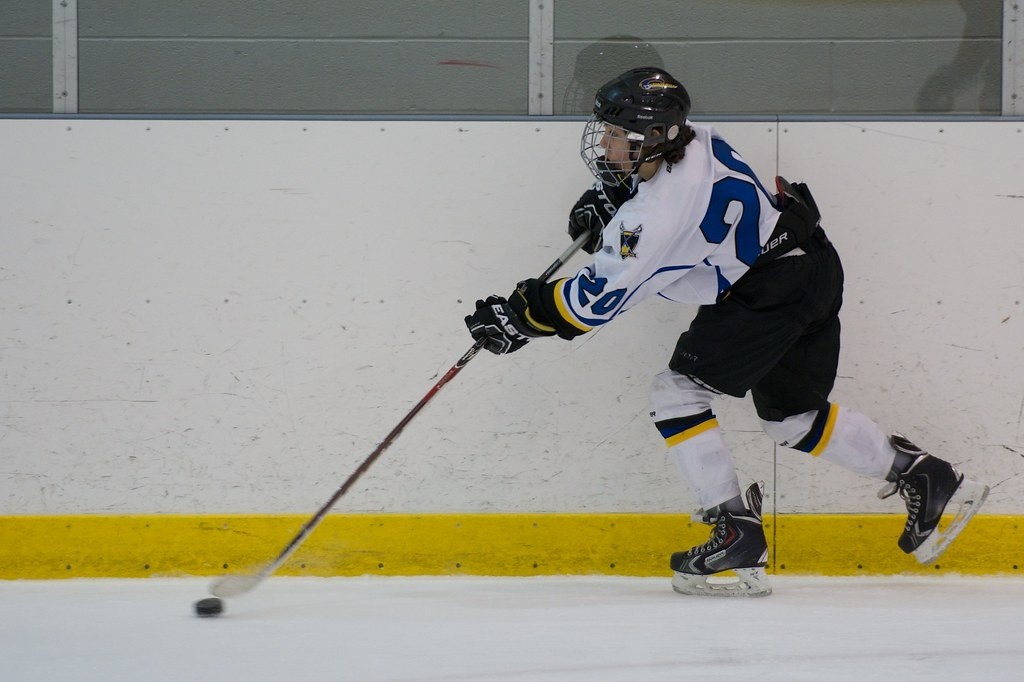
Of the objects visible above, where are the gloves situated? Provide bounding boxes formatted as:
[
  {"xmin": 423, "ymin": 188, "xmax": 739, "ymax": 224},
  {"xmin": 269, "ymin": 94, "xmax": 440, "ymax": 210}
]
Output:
[
  {"xmin": 464, "ymin": 278, "xmax": 557, "ymax": 355},
  {"xmin": 568, "ymin": 180, "xmax": 627, "ymax": 254}
]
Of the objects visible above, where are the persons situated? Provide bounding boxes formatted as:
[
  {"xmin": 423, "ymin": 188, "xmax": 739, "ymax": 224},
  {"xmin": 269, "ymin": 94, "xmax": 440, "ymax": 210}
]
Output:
[{"xmin": 464, "ymin": 67, "xmax": 990, "ymax": 598}]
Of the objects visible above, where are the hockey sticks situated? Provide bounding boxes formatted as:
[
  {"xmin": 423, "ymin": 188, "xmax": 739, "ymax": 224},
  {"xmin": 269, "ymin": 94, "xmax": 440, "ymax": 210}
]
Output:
[{"xmin": 210, "ymin": 228, "xmax": 593, "ymax": 598}]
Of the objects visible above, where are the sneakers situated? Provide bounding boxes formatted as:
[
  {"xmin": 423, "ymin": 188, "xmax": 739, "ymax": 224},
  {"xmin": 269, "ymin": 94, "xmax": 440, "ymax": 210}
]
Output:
[
  {"xmin": 877, "ymin": 431, "xmax": 989, "ymax": 564},
  {"xmin": 671, "ymin": 480, "xmax": 773, "ymax": 597}
]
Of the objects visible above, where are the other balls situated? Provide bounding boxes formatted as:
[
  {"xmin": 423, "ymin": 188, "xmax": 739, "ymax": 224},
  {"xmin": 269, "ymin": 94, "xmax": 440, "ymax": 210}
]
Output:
[{"xmin": 195, "ymin": 598, "xmax": 223, "ymax": 617}]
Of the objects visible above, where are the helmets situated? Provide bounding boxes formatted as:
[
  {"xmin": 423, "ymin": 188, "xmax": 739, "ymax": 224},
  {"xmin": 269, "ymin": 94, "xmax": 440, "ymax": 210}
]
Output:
[{"xmin": 593, "ymin": 67, "xmax": 691, "ymax": 135}]
